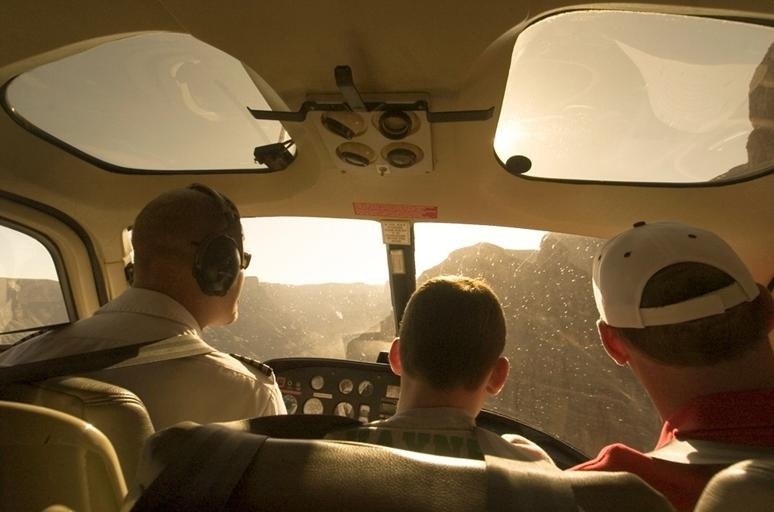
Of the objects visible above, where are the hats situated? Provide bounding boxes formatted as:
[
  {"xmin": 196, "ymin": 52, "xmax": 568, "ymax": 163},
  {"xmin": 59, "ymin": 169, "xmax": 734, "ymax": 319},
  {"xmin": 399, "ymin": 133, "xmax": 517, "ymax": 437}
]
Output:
[{"xmin": 592, "ymin": 220, "xmax": 760, "ymax": 329}]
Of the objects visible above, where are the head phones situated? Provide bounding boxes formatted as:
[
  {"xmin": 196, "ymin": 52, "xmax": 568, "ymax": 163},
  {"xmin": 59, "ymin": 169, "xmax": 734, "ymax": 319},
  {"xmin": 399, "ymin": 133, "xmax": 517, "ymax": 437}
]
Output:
[{"xmin": 121, "ymin": 182, "xmax": 241, "ymax": 296}]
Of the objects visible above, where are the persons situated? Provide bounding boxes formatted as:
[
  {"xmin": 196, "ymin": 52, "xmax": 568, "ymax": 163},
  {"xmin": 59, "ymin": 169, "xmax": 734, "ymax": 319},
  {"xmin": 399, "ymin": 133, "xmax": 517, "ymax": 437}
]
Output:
[
  {"xmin": 321, "ymin": 274, "xmax": 561, "ymax": 468},
  {"xmin": 0, "ymin": 183, "xmax": 289, "ymax": 428},
  {"xmin": 565, "ymin": 223, "xmax": 774, "ymax": 511}
]
